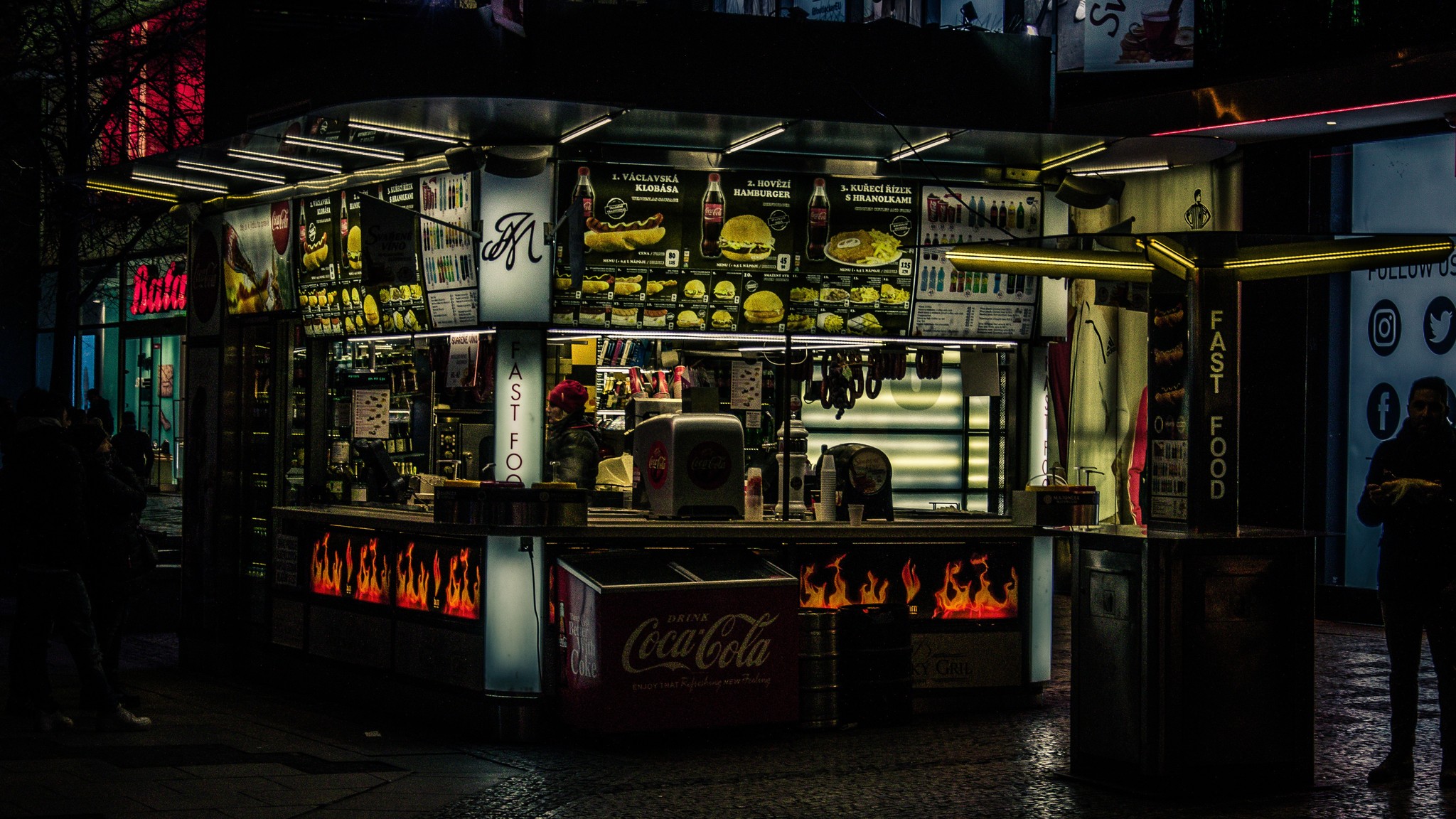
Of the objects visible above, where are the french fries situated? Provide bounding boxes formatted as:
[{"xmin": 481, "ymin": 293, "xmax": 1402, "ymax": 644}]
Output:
[
  {"xmin": 824, "ymin": 315, "xmax": 844, "ymax": 333},
  {"xmin": 860, "ymin": 286, "xmax": 878, "ymax": 302},
  {"xmin": 799, "ymin": 287, "xmax": 819, "ymax": 302},
  {"xmin": 390, "ymin": 284, "xmax": 422, "ymax": 300},
  {"xmin": 855, "ymin": 229, "xmax": 903, "ymax": 264},
  {"xmin": 885, "ymin": 290, "xmax": 910, "ymax": 304}
]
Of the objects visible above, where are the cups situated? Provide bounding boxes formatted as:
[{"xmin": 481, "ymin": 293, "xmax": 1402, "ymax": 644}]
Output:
[
  {"xmin": 848, "ymin": 504, "xmax": 864, "ymax": 526},
  {"xmin": 927, "ymin": 197, "xmax": 941, "ymax": 223},
  {"xmin": 937, "ymin": 201, "xmax": 949, "ymax": 223},
  {"xmin": 628, "ymin": 366, "xmax": 649, "ymax": 399},
  {"xmin": 814, "ymin": 503, "xmax": 821, "ymax": 522},
  {"xmin": 745, "ymin": 467, "xmax": 763, "ymax": 521},
  {"xmin": 651, "ymin": 371, "xmax": 670, "ymax": 399},
  {"xmin": 947, "ymin": 208, "xmax": 956, "ymax": 223},
  {"xmin": 820, "ymin": 455, "xmax": 836, "ymax": 522},
  {"xmin": 673, "ymin": 365, "xmax": 685, "ymax": 399}
]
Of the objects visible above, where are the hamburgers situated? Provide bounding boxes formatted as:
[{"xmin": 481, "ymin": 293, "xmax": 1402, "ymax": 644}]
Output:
[
  {"xmin": 346, "ymin": 225, "xmax": 363, "ymax": 269},
  {"xmin": 714, "ymin": 281, "xmax": 735, "ymax": 299},
  {"xmin": 719, "ymin": 215, "xmax": 776, "ymax": 262},
  {"xmin": 676, "ymin": 310, "xmax": 705, "ymax": 327},
  {"xmin": 712, "ymin": 310, "xmax": 733, "ymax": 327},
  {"xmin": 342, "ymin": 288, "xmax": 379, "ymax": 332},
  {"xmin": 683, "ymin": 280, "xmax": 706, "ymax": 298},
  {"xmin": 880, "ymin": 284, "xmax": 895, "ymax": 301},
  {"xmin": 743, "ymin": 290, "xmax": 783, "ymax": 323}
]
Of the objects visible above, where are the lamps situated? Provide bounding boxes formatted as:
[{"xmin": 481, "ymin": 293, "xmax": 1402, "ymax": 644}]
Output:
[
  {"xmin": 82, "ymin": 122, "xmax": 472, "ymax": 203},
  {"xmin": 723, "ymin": 125, "xmax": 785, "ymax": 154},
  {"xmin": 1041, "ymin": 145, "xmax": 1107, "ymax": 171},
  {"xmin": 885, "ymin": 134, "xmax": 951, "ymax": 162},
  {"xmin": 558, "ymin": 117, "xmax": 612, "ymax": 144},
  {"xmin": 1072, "ymin": 164, "xmax": 1174, "ymax": 177},
  {"xmin": 943, "ymin": 235, "xmax": 1456, "ymax": 283}
]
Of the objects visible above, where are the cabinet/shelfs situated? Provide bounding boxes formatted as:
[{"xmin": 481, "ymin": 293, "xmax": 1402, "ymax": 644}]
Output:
[{"xmin": 287, "ymin": 388, "xmax": 425, "ymax": 487}]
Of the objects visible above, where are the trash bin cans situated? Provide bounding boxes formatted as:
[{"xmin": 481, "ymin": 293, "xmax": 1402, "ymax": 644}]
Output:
[{"xmin": 1048, "ymin": 521, "xmax": 1322, "ymax": 782}]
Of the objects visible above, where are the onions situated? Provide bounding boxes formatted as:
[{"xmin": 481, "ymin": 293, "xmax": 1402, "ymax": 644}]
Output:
[{"xmin": 837, "ymin": 238, "xmax": 860, "ymax": 248}]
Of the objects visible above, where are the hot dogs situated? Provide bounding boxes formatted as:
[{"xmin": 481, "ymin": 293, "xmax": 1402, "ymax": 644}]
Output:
[
  {"xmin": 556, "ymin": 271, "xmax": 677, "ymax": 296},
  {"xmin": 303, "ymin": 231, "xmax": 328, "ymax": 269},
  {"xmin": 583, "ymin": 213, "xmax": 665, "ymax": 251}
]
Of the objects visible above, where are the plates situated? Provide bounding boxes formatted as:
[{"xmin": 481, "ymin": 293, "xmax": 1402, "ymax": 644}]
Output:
[
  {"xmin": 824, "ymin": 242, "xmax": 903, "ymax": 267},
  {"xmin": 790, "ymin": 288, "xmax": 910, "ymax": 305}
]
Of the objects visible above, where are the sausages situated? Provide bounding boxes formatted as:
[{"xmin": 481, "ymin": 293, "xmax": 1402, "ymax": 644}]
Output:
[{"xmin": 612, "ymin": 308, "xmax": 667, "ymax": 317}]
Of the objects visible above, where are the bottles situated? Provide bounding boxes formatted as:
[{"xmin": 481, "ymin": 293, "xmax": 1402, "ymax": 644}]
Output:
[
  {"xmin": 340, "ymin": 192, "xmax": 350, "ymax": 269},
  {"xmin": 968, "ymin": 196, "xmax": 976, "ymax": 227},
  {"xmin": 949, "ymin": 266, "xmax": 1034, "ymax": 295},
  {"xmin": 700, "ymin": 172, "xmax": 725, "ymax": 257},
  {"xmin": 378, "ymin": 184, "xmax": 384, "ymax": 201},
  {"xmin": 1030, "ymin": 203, "xmax": 1037, "ymax": 231},
  {"xmin": 990, "ymin": 200, "xmax": 998, "ymax": 227},
  {"xmin": 999, "ymin": 201, "xmax": 1007, "ymax": 228},
  {"xmin": 299, "ymin": 199, "xmax": 307, "ymax": 274},
  {"xmin": 1016, "ymin": 202, "xmax": 1025, "ymax": 229},
  {"xmin": 222, "ymin": 219, "xmax": 255, "ymax": 282},
  {"xmin": 977, "ymin": 197, "xmax": 985, "ymax": 227},
  {"xmin": 921, "ymin": 266, "xmax": 945, "ymax": 292},
  {"xmin": 247, "ymin": 349, "xmax": 418, "ymax": 579},
  {"xmin": 1008, "ymin": 201, "xmax": 1016, "ymax": 229},
  {"xmin": 422, "ymin": 177, "xmax": 473, "ymax": 285},
  {"xmin": 923, "ymin": 233, "xmax": 993, "ymax": 261},
  {"xmin": 572, "ymin": 167, "xmax": 595, "ymax": 253},
  {"xmin": 806, "ymin": 178, "xmax": 830, "ymax": 261}
]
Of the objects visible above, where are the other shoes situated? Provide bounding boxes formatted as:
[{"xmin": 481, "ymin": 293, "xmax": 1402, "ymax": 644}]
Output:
[
  {"xmin": 115, "ymin": 703, "xmax": 153, "ymax": 729},
  {"xmin": 1439, "ymin": 771, "xmax": 1455, "ymax": 791},
  {"xmin": 1367, "ymin": 754, "xmax": 1415, "ymax": 788},
  {"xmin": 43, "ymin": 711, "xmax": 74, "ymax": 730}
]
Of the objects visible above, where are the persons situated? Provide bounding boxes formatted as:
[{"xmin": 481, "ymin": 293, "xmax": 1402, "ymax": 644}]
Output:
[
  {"xmin": 545, "ymin": 380, "xmax": 599, "ymax": 489},
  {"xmin": 0, "ymin": 387, "xmax": 155, "ymax": 733},
  {"xmin": 1357, "ymin": 376, "xmax": 1456, "ymax": 793}
]
